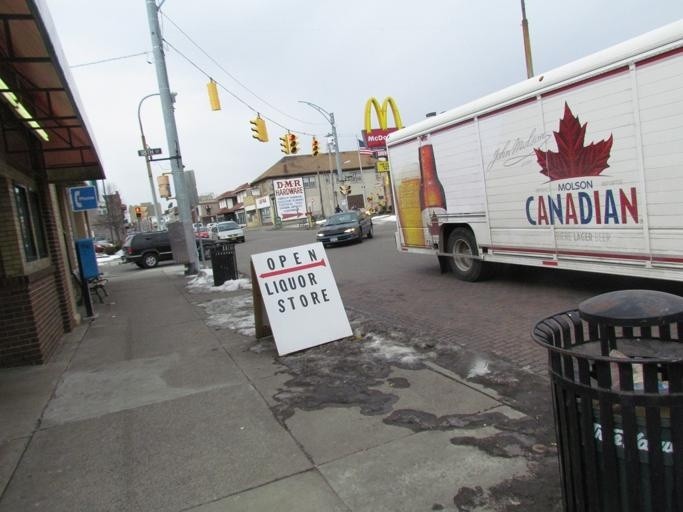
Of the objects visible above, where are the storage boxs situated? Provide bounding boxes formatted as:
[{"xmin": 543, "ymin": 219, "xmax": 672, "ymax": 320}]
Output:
[{"xmin": 72, "ymin": 268, "xmax": 109, "ymax": 306}]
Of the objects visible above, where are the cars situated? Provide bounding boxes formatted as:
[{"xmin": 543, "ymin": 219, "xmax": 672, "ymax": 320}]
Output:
[{"xmin": 392, "ymin": 162, "xmax": 420, "ymax": 247}]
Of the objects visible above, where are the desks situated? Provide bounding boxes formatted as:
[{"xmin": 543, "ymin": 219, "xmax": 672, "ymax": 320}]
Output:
[{"xmin": 137, "ymin": 92, "xmax": 180, "ymax": 230}]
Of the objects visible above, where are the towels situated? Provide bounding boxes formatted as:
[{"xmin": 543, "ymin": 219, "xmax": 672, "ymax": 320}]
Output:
[
  {"xmin": 211, "ymin": 240, "xmax": 238, "ymax": 286},
  {"xmin": 532, "ymin": 291, "xmax": 683, "ymax": 512}
]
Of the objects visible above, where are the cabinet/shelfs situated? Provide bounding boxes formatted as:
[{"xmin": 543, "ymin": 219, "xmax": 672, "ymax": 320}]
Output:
[{"xmin": 417, "ymin": 133, "xmax": 448, "ymax": 249}]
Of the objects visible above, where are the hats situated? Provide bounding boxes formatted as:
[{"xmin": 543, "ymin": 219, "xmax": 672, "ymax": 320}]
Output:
[
  {"xmin": 122, "ymin": 231, "xmax": 214, "ymax": 270},
  {"xmin": 192, "ymin": 220, "xmax": 245, "ymax": 245},
  {"xmin": 316, "ymin": 209, "xmax": 373, "ymax": 248},
  {"xmin": 93, "ymin": 239, "xmax": 115, "ymax": 254}
]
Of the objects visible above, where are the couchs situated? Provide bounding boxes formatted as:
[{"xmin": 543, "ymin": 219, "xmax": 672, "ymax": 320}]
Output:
[
  {"xmin": 134, "ymin": 207, "xmax": 148, "ymax": 220},
  {"xmin": 279, "ymin": 132, "xmax": 299, "ymax": 155},
  {"xmin": 338, "ymin": 185, "xmax": 353, "ymax": 196},
  {"xmin": 250, "ymin": 113, "xmax": 268, "ymax": 143},
  {"xmin": 312, "ymin": 135, "xmax": 319, "ymax": 157}
]
